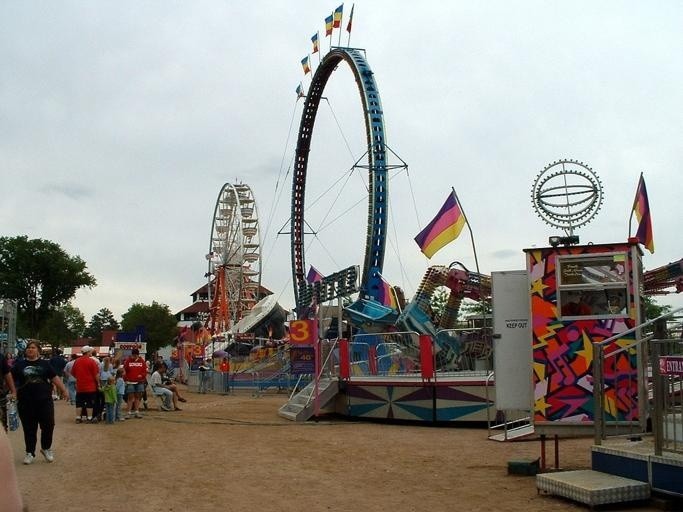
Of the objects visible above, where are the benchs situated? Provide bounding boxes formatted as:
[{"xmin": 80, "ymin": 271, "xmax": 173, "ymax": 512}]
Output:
[{"xmin": 144, "ymin": 372, "xmax": 174, "ymax": 413}]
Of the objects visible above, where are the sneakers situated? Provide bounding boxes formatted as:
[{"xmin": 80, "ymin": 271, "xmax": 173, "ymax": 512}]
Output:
[
  {"xmin": 160, "ymin": 405, "xmax": 170, "ymax": 411},
  {"xmin": 125, "ymin": 413, "xmax": 143, "ymax": 418},
  {"xmin": 75, "ymin": 418, "xmax": 100, "ymax": 424},
  {"xmin": 22, "ymin": 452, "xmax": 34, "ymax": 464},
  {"xmin": 40, "ymin": 448, "xmax": 55, "ymax": 462}
]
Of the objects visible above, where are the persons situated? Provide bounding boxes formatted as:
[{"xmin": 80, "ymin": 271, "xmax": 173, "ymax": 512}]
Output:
[
  {"xmin": 220, "ymin": 357, "xmax": 230, "ymax": 391},
  {"xmin": 1, "ymin": 339, "xmax": 187, "ymax": 512},
  {"xmin": 197, "ymin": 359, "xmax": 211, "ymax": 394}
]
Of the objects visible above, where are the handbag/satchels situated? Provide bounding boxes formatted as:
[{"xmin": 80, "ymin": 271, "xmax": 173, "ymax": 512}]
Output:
[{"xmin": 6, "ymin": 400, "xmax": 21, "ymax": 433}]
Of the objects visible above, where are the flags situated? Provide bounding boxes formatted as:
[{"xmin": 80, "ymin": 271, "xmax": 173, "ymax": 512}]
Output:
[
  {"xmin": 306, "ymin": 266, "xmax": 323, "ymax": 284},
  {"xmin": 295, "ymin": 85, "xmax": 301, "ymax": 96},
  {"xmin": 301, "ymin": 56, "xmax": 310, "ymax": 75},
  {"xmin": 377, "ymin": 280, "xmax": 398, "ymax": 311},
  {"xmin": 311, "ymin": 33, "xmax": 319, "ymax": 54},
  {"xmin": 325, "ymin": 14, "xmax": 333, "ymax": 37},
  {"xmin": 633, "ymin": 175, "xmax": 654, "ymax": 254},
  {"xmin": 413, "ymin": 190, "xmax": 466, "ymax": 259},
  {"xmin": 346, "ymin": 5, "xmax": 356, "ymax": 33},
  {"xmin": 332, "ymin": 4, "xmax": 343, "ymax": 28}
]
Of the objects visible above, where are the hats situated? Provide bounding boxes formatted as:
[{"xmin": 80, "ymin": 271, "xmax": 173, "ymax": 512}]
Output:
[{"xmin": 81, "ymin": 345, "xmax": 94, "ymax": 353}]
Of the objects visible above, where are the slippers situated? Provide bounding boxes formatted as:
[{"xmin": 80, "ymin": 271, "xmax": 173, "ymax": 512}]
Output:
[
  {"xmin": 175, "ymin": 407, "xmax": 183, "ymax": 412},
  {"xmin": 177, "ymin": 397, "xmax": 187, "ymax": 402}
]
